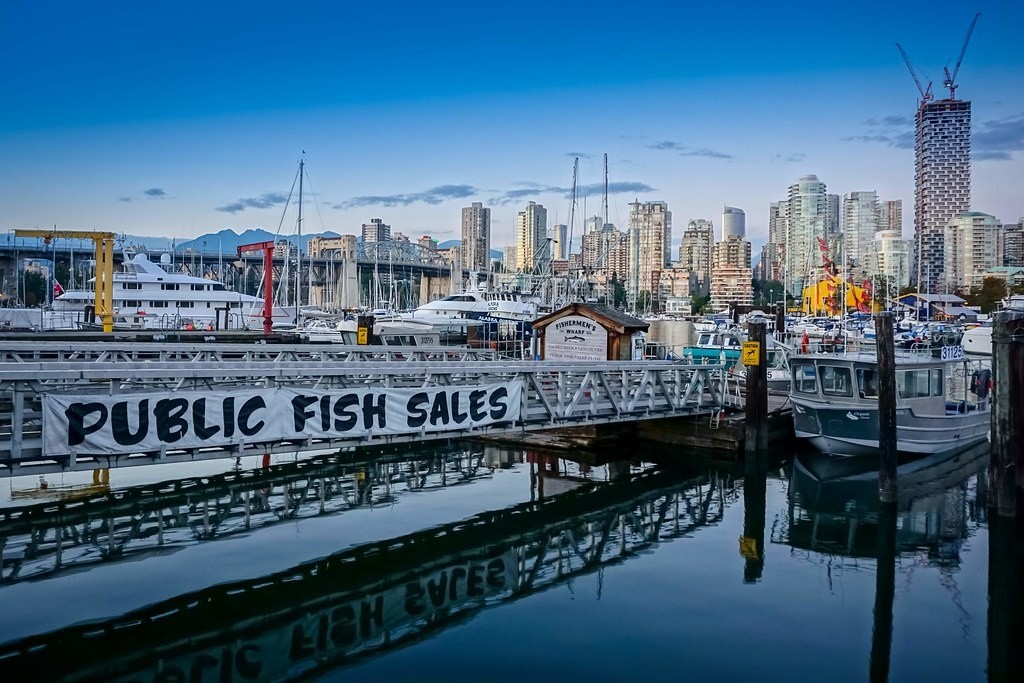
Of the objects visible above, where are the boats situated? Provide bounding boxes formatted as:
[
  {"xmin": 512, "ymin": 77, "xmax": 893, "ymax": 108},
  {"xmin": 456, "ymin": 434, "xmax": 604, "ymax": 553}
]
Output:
[
  {"xmin": 685, "ymin": 293, "xmax": 1023, "ymax": 375},
  {"xmin": 3, "ymin": 246, "xmax": 687, "ymax": 366},
  {"xmin": 787, "ymin": 344, "xmax": 994, "ymax": 458},
  {"xmin": 707, "ymin": 330, "xmax": 798, "ymax": 431}
]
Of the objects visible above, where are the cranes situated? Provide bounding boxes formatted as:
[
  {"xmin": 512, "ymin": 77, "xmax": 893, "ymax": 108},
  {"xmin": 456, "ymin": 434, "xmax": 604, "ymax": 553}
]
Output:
[
  {"xmin": 943, "ymin": 11, "xmax": 983, "ymax": 101},
  {"xmin": 894, "ymin": 41, "xmax": 934, "ymax": 110}
]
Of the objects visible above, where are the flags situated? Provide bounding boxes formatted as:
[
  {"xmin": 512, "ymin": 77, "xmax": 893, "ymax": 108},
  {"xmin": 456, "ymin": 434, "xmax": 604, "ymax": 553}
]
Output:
[
  {"xmin": 54, "ymin": 278, "xmax": 65, "ymax": 300},
  {"xmin": 850, "ymin": 273, "xmax": 873, "ymax": 314},
  {"xmin": 816, "ymin": 235, "xmax": 837, "ymax": 316}
]
